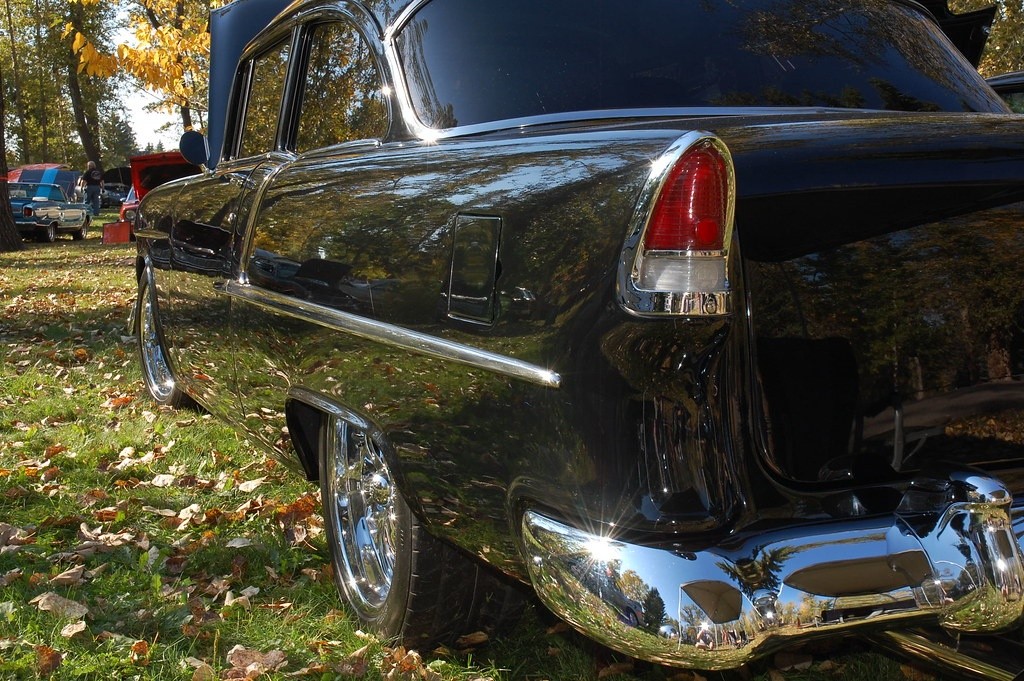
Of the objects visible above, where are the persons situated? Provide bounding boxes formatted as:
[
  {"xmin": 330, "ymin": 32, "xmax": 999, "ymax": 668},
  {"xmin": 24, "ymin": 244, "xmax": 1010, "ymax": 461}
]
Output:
[{"xmin": 81, "ymin": 161, "xmax": 105, "ymax": 216}]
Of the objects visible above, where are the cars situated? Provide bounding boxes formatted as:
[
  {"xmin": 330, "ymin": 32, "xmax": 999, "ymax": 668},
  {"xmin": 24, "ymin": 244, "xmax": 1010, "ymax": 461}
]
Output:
[
  {"xmin": 4, "ymin": 162, "xmax": 85, "ymax": 202},
  {"xmin": 143, "ymin": 213, "xmax": 537, "ymax": 327},
  {"xmin": 119, "ymin": 150, "xmax": 203, "ymax": 241},
  {"xmin": 7, "ymin": 168, "xmax": 94, "ymax": 243},
  {"xmin": 135, "ymin": 0, "xmax": 1024, "ymax": 681},
  {"xmin": 98, "ymin": 167, "xmax": 133, "ymax": 207}
]
